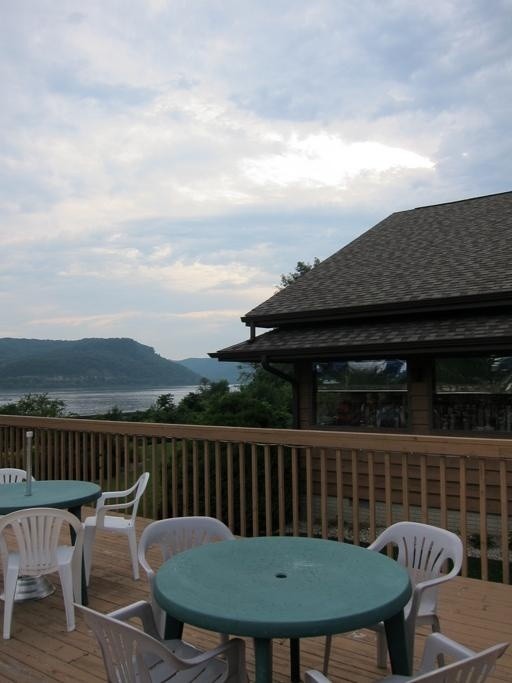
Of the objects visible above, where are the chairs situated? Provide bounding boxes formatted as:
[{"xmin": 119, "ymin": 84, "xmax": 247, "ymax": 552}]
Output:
[{"xmin": 0, "ymin": 467, "xmax": 150, "ymax": 639}]
